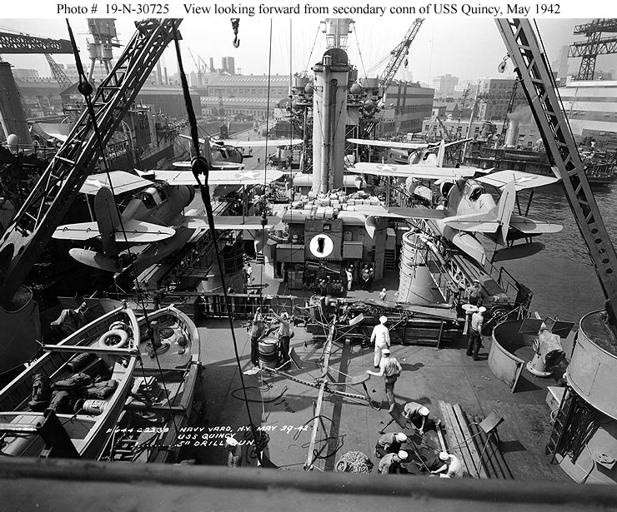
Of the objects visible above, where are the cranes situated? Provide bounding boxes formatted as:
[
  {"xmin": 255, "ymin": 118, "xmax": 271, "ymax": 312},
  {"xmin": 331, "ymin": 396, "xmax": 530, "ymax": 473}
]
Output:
[
  {"xmin": 0, "ymin": 24, "xmax": 80, "ymax": 55},
  {"xmin": 494, "ymin": 15, "xmax": 617, "ymax": 464},
  {"xmin": 355, "ymin": 12, "xmax": 424, "ymax": 127},
  {"xmin": 0, "ymin": 20, "xmax": 179, "ymax": 293}
]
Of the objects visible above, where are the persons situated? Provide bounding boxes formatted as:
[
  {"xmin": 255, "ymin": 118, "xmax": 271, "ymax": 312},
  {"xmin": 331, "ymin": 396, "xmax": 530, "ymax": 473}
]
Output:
[
  {"xmin": 366, "ymin": 348, "xmax": 402, "ymax": 413},
  {"xmin": 316, "ymin": 263, "xmax": 374, "ymax": 297},
  {"xmin": 269, "ymin": 309, "xmax": 291, "ymax": 371},
  {"xmin": 375, "ymin": 431, "xmax": 407, "ymax": 457},
  {"xmin": 251, "ymin": 307, "xmax": 265, "ymax": 367},
  {"xmin": 379, "ymin": 287, "xmax": 387, "ymax": 301},
  {"xmin": 403, "ymin": 402, "xmax": 430, "ymax": 435},
  {"xmin": 370, "ymin": 316, "xmax": 391, "ymax": 368},
  {"xmin": 466, "ymin": 306, "xmax": 486, "ymax": 360},
  {"xmin": 430, "ymin": 452, "xmax": 463, "ymax": 479},
  {"xmin": 378, "ymin": 450, "xmax": 409, "ymax": 475},
  {"xmin": 224, "ymin": 437, "xmax": 241, "ymax": 467}
]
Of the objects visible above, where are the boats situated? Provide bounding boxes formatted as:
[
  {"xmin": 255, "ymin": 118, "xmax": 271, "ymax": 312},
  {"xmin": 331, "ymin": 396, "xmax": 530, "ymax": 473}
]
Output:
[
  {"xmin": 0, "ymin": 297, "xmax": 140, "ymax": 457},
  {"xmin": 97, "ymin": 301, "xmax": 201, "ymax": 462}
]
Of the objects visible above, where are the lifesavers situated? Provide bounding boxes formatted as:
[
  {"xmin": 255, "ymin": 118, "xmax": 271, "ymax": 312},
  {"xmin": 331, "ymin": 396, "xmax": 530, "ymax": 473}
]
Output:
[{"xmin": 99, "ymin": 329, "xmax": 128, "ymax": 349}]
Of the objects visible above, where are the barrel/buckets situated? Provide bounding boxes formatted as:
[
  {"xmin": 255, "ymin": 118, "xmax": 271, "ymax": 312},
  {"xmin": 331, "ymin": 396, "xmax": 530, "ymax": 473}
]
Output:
[{"xmin": 258, "ymin": 337, "xmax": 279, "ymax": 370}]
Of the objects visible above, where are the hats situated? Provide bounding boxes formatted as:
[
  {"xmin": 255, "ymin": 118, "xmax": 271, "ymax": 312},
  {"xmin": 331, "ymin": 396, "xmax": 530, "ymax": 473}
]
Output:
[
  {"xmin": 479, "ymin": 306, "xmax": 487, "ymax": 312},
  {"xmin": 397, "ymin": 432, "xmax": 407, "ymax": 442},
  {"xmin": 419, "ymin": 407, "xmax": 430, "ymax": 416},
  {"xmin": 398, "ymin": 450, "xmax": 408, "ymax": 460},
  {"xmin": 382, "ymin": 348, "xmax": 390, "ymax": 355},
  {"xmin": 439, "ymin": 452, "xmax": 449, "ymax": 460},
  {"xmin": 379, "ymin": 315, "xmax": 387, "ymax": 323}
]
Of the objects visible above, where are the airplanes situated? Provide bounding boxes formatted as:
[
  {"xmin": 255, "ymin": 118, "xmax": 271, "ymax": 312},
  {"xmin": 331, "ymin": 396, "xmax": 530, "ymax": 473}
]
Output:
[
  {"xmin": 346, "ymin": 159, "xmax": 563, "ymax": 246},
  {"xmin": 51, "ymin": 167, "xmax": 282, "ymax": 273},
  {"xmin": 172, "ymin": 134, "xmax": 304, "ymax": 168},
  {"xmin": 346, "ymin": 136, "xmax": 474, "ymax": 168}
]
